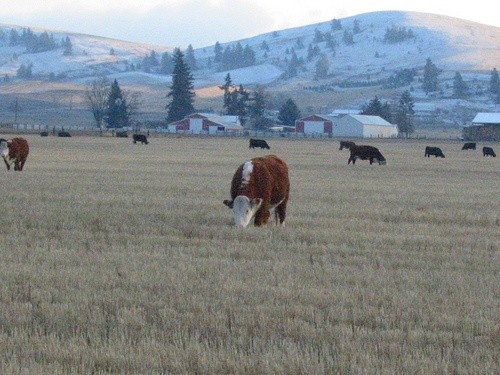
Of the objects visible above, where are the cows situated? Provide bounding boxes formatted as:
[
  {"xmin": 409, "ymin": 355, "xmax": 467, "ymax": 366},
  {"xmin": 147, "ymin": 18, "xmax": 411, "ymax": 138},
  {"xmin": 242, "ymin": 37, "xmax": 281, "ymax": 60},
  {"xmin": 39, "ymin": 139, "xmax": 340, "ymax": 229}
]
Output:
[
  {"xmin": 222, "ymin": 154, "xmax": 292, "ymax": 229},
  {"xmin": 132, "ymin": 134, "xmax": 150, "ymax": 145},
  {"xmin": 58, "ymin": 132, "xmax": 72, "ymax": 137},
  {"xmin": 482, "ymin": 146, "xmax": 496, "ymax": 158},
  {"xmin": 424, "ymin": 145, "xmax": 445, "ymax": 159},
  {"xmin": 461, "ymin": 142, "xmax": 477, "ymax": 151},
  {"xmin": 40, "ymin": 132, "xmax": 48, "ymax": 136},
  {"xmin": 248, "ymin": 137, "xmax": 270, "ymax": 151},
  {"xmin": 0, "ymin": 137, "xmax": 29, "ymax": 172},
  {"xmin": 115, "ymin": 130, "xmax": 129, "ymax": 138},
  {"xmin": 338, "ymin": 140, "xmax": 386, "ymax": 166}
]
